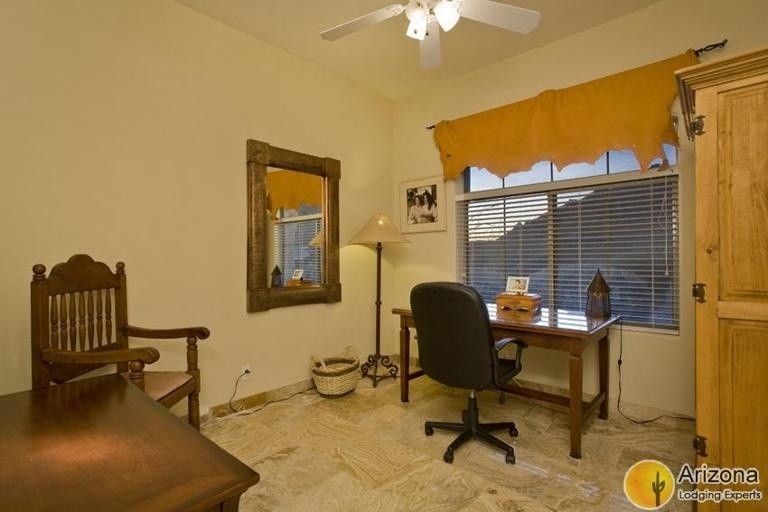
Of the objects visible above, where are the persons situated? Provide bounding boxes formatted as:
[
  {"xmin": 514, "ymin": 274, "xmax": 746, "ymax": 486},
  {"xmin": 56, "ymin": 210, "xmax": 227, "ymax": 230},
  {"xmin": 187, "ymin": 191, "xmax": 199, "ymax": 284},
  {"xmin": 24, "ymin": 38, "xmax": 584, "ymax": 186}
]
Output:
[
  {"xmin": 405, "ymin": 194, "xmax": 423, "ymax": 223},
  {"xmin": 511, "ymin": 279, "xmax": 525, "ymax": 290},
  {"xmin": 421, "ymin": 191, "xmax": 437, "ymax": 222}
]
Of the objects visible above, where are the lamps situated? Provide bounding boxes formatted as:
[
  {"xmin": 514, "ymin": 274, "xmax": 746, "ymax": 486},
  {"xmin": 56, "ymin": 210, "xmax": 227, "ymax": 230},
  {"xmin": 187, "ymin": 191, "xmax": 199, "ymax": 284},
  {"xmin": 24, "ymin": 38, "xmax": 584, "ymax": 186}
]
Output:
[
  {"xmin": 406, "ymin": 3, "xmax": 462, "ymax": 43},
  {"xmin": 345, "ymin": 213, "xmax": 413, "ymax": 391}
]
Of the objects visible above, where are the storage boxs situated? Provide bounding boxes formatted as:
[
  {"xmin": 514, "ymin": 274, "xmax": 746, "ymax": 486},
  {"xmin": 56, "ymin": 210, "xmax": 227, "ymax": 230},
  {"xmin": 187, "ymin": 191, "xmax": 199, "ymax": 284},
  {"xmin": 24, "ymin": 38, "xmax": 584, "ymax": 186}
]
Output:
[{"xmin": 497, "ymin": 292, "xmax": 542, "ymax": 322}]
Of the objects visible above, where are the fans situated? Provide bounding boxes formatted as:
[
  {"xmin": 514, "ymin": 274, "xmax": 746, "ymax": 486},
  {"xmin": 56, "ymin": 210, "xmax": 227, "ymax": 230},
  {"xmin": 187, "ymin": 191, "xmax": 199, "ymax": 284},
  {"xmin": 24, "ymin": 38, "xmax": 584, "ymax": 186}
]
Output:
[{"xmin": 318, "ymin": 3, "xmax": 542, "ymax": 70}]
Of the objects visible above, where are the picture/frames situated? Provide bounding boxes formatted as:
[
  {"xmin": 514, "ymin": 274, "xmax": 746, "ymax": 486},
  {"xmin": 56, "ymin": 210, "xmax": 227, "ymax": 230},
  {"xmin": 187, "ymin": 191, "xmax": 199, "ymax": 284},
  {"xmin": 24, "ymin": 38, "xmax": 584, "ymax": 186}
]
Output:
[{"xmin": 507, "ymin": 275, "xmax": 530, "ymax": 294}]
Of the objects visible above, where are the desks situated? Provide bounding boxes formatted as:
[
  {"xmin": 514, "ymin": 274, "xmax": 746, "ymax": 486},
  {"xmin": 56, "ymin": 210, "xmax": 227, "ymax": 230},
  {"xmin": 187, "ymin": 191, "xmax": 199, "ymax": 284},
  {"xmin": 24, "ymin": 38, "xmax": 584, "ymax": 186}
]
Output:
[
  {"xmin": 0, "ymin": 372, "xmax": 260, "ymax": 511},
  {"xmin": 389, "ymin": 303, "xmax": 624, "ymax": 459}
]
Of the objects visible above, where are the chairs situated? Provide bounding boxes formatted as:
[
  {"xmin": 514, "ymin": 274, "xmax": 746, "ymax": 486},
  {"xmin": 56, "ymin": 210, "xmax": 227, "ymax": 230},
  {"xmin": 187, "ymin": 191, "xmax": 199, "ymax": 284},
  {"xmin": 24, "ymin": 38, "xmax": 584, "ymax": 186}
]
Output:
[
  {"xmin": 410, "ymin": 281, "xmax": 527, "ymax": 462},
  {"xmin": 30, "ymin": 254, "xmax": 210, "ymax": 433}
]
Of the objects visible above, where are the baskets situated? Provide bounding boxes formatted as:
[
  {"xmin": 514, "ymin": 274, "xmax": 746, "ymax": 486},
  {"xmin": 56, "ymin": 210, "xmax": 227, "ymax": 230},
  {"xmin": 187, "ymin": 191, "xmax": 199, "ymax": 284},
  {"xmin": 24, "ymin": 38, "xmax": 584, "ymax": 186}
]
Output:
[{"xmin": 312, "ymin": 345, "xmax": 360, "ymax": 396}]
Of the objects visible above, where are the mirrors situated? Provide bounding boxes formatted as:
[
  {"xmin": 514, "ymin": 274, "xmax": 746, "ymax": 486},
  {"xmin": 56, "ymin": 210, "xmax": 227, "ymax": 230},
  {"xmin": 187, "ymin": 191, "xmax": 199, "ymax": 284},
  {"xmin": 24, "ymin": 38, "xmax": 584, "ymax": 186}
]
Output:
[{"xmin": 245, "ymin": 138, "xmax": 341, "ymax": 314}]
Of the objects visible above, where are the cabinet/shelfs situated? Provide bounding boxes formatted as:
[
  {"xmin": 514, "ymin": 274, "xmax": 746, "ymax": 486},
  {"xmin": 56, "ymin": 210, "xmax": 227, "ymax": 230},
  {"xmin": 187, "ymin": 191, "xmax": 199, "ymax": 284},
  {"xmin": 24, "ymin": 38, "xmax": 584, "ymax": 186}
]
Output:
[{"xmin": 671, "ymin": 43, "xmax": 768, "ymax": 509}]
